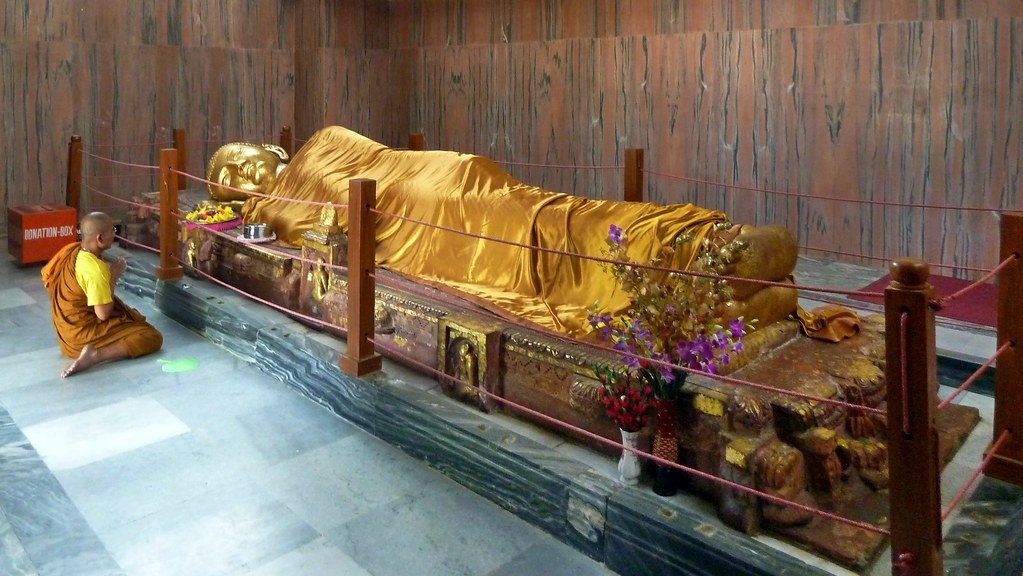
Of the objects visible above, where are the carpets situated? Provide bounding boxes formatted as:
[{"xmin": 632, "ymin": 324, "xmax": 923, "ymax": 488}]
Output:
[{"xmin": 845, "ymin": 270, "xmax": 999, "ymax": 329}]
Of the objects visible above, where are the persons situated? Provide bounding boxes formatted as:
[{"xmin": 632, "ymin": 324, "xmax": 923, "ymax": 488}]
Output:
[
  {"xmin": 43, "ymin": 211, "xmax": 163, "ymax": 380},
  {"xmin": 207, "ymin": 126, "xmax": 796, "ymax": 339}
]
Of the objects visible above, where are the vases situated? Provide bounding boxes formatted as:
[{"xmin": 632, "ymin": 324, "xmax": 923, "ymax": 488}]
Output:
[
  {"xmin": 618, "ymin": 428, "xmax": 643, "ymax": 485},
  {"xmin": 653, "ymin": 397, "xmax": 680, "ymax": 497}
]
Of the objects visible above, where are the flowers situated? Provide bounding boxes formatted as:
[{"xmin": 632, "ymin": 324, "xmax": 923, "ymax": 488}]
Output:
[{"xmin": 586, "ymin": 223, "xmax": 760, "ymax": 433}]
